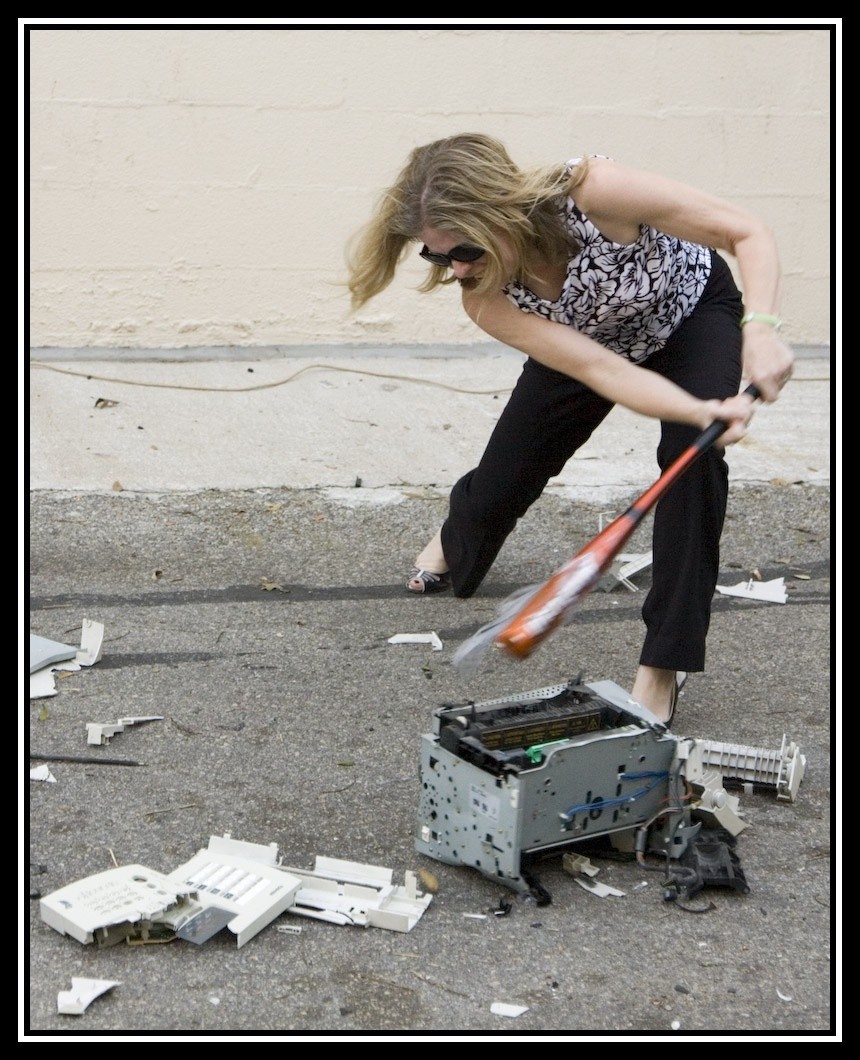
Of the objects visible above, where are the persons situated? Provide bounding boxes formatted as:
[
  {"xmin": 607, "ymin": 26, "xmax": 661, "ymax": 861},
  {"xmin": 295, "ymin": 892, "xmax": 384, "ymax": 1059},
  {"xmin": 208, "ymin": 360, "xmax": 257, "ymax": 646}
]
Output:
[{"xmin": 344, "ymin": 132, "xmax": 795, "ymax": 728}]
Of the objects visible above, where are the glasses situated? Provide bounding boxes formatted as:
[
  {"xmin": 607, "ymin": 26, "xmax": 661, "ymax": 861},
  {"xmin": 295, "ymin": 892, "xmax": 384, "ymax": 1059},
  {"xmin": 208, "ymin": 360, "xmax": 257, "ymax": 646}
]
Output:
[{"xmin": 419, "ymin": 239, "xmax": 488, "ymax": 268}]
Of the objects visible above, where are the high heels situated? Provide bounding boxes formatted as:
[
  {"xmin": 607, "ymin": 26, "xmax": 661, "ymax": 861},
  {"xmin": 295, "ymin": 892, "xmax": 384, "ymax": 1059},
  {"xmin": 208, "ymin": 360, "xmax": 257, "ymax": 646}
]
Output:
[
  {"xmin": 402, "ymin": 564, "xmax": 454, "ymax": 593},
  {"xmin": 649, "ymin": 671, "xmax": 690, "ymax": 726}
]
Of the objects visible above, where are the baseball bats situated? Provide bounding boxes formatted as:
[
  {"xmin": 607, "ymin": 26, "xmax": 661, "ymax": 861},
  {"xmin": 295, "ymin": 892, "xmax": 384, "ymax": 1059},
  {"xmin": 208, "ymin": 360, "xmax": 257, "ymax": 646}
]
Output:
[{"xmin": 499, "ymin": 384, "xmax": 763, "ymax": 661}]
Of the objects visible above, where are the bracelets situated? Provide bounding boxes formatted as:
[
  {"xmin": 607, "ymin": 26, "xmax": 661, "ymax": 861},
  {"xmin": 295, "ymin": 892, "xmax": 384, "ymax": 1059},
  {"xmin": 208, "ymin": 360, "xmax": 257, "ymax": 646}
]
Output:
[{"xmin": 740, "ymin": 314, "xmax": 783, "ymax": 330}]
